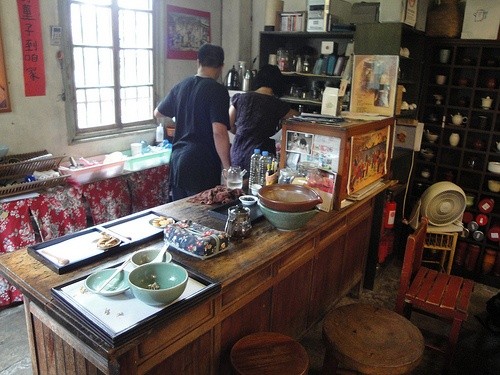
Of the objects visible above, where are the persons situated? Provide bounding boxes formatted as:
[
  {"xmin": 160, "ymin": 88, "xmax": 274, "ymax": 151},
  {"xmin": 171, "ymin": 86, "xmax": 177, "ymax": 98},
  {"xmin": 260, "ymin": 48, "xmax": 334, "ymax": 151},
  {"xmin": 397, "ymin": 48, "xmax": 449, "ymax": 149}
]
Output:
[
  {"xmin": 289, "ymin": 133, "xmax": 309, "ymax": 153},
  {"xmin": 228, "ymin": 63, "xmax": 301, "ymax": 179},
  {"xmin": 153, "ymin": 43, "xmax": 231, "ymax": 203}
]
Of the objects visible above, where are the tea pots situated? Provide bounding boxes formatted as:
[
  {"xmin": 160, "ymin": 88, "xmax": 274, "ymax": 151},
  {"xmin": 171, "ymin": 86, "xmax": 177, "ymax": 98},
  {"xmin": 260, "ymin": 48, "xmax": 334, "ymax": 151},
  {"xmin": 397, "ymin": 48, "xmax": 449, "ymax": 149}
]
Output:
[{"xmin": 450, "ymin": 112, "xmax": 469, "ymax": 125}]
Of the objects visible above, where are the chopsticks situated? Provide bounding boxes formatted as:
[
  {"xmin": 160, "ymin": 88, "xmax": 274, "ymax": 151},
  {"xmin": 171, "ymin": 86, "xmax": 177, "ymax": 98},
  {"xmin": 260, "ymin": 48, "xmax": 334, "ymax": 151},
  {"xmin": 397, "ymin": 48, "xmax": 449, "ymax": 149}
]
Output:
[{"xmin": 95, "ymin": 259, "xmax": 132, "ymax": 294}]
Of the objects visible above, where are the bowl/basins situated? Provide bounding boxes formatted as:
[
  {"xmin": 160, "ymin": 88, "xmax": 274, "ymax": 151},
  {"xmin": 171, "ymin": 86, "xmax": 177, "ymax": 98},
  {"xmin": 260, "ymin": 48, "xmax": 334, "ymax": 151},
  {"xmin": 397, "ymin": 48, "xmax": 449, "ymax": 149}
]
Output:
[
  {"xmin": 131, "ymin": 250, "xmax": 172, "ymax": 268},
  {"xmin": 128, "ymin": 262, "xmax": 188, "ymax": 309},
  {"xmin": 257, "ymin": 200, "xmax": 319, "ymax": 231},
  {"xmin": 256, "ymin": 184, "xmax": 323, "ymax": 213}
]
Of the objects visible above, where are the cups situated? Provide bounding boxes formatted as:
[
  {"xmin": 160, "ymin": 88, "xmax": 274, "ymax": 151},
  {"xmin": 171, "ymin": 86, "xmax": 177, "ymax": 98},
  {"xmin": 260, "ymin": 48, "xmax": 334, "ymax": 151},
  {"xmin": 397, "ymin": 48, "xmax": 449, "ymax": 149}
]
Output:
[{"xmin": 421, "ymin": 49, "xmax": 500, "ymax": 193}]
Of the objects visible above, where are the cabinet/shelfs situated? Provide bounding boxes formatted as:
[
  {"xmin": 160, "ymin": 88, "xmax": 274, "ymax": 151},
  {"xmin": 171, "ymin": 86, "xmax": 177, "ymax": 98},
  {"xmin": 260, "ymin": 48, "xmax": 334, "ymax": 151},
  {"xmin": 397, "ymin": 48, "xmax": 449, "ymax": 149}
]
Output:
[{"xmin": 259, "ymin": 23, "xmax": 500, "ymax": 251}]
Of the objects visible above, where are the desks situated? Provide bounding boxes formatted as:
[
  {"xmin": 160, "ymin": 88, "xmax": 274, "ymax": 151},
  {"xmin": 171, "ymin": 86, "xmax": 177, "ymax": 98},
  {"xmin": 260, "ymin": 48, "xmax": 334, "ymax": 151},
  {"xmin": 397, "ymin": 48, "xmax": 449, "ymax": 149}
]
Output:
[
  {"xmin": 419, "ymin": 230, "xmax": 458, "ymax": 274},
  {"xmin": 0, "ymin": 165, "xmax": 168, "ymax": 311}
]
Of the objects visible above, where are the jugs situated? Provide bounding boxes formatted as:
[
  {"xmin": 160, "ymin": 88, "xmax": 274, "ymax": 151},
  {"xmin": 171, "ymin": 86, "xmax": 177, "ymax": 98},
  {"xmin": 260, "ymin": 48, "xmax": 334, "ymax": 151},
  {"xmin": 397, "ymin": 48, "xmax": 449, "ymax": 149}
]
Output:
[
  {"xmin": 225, "ymin": 206, "xmax": 253, "ymax": 241},
  {"xmin": 221, "ymin": 165, "xmax": 247, "ymax": 190}
]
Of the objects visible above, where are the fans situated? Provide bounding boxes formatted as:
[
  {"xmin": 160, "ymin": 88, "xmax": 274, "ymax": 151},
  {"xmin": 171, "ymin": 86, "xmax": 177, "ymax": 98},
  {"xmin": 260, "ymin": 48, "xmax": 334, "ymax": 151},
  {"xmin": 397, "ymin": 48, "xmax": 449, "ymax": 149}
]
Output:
[{"xmin": 410, "ymin": 182, "xmax": 467, "ymax": 233}]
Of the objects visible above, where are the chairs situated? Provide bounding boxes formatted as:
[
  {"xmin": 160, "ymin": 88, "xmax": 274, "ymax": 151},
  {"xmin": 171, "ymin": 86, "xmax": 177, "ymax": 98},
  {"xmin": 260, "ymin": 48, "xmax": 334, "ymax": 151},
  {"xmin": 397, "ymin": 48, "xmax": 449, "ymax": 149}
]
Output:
[{"xmin": 394, "ymin": 218, "xmax": 475, "ymax": 361}]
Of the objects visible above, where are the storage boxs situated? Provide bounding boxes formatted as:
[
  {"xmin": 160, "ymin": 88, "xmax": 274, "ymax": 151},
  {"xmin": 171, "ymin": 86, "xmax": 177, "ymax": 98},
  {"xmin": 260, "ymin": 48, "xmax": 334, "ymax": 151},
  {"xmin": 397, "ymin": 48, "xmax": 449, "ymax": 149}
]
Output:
[{"xmin": 62, "ymin": 146, "xmax": 173, "ymax": 184}]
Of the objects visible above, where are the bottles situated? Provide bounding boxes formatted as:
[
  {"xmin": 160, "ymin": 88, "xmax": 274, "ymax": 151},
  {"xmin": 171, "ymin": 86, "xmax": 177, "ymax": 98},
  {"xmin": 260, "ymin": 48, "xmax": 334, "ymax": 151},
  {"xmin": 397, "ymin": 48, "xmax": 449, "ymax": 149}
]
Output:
[
  {"xmin": 450, "ymin": 197, "xmax": 500, "ymax": 279},
  {"xmin": 263, "ymin": 45, "xmax": 346, "ymax": 76},
  {"xmin": 249, "ymin": 149, "xmax": 262, "ymax": 189},
  {"xmin": 258, "ymin": 151, "xmax": 271, "ymax": 186}
]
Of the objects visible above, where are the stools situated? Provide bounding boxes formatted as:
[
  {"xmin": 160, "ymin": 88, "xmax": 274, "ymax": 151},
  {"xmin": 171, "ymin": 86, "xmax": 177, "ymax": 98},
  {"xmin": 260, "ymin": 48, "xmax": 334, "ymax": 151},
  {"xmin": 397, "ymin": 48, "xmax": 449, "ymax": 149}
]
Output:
[
  {"xmin": 230, "ymin": 332, "xmax": 309, "ymax": 375},
  {"xmin": 323, "ymin": 303, "xmax": 426, "ymax": 375}
]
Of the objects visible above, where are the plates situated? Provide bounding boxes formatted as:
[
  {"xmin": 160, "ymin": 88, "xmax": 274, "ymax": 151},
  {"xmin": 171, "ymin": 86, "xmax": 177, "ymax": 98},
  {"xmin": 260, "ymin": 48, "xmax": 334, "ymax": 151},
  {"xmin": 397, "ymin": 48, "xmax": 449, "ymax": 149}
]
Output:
[
  {"xmin": 91, "ymin": 237, "xmax": 122, "ymax": 250},
  {"xmin": 148, "ymin": 217, "xmax": 176, "ymax": 230},
  {"xmin": 238, "ymin": 195, "xmax": 259, "ymax": 206},
  {"xmin": 85, "ymin": 269, "xmax": 131, "ymax": 295}
]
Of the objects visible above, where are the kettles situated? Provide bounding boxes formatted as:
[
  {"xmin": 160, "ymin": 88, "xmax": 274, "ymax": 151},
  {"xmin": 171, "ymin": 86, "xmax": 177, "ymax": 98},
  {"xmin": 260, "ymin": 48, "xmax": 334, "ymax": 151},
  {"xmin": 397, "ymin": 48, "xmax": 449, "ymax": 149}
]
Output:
[{"xmin": 223, "ymin": 70, "xmax": 240, "ymax": 90}]
